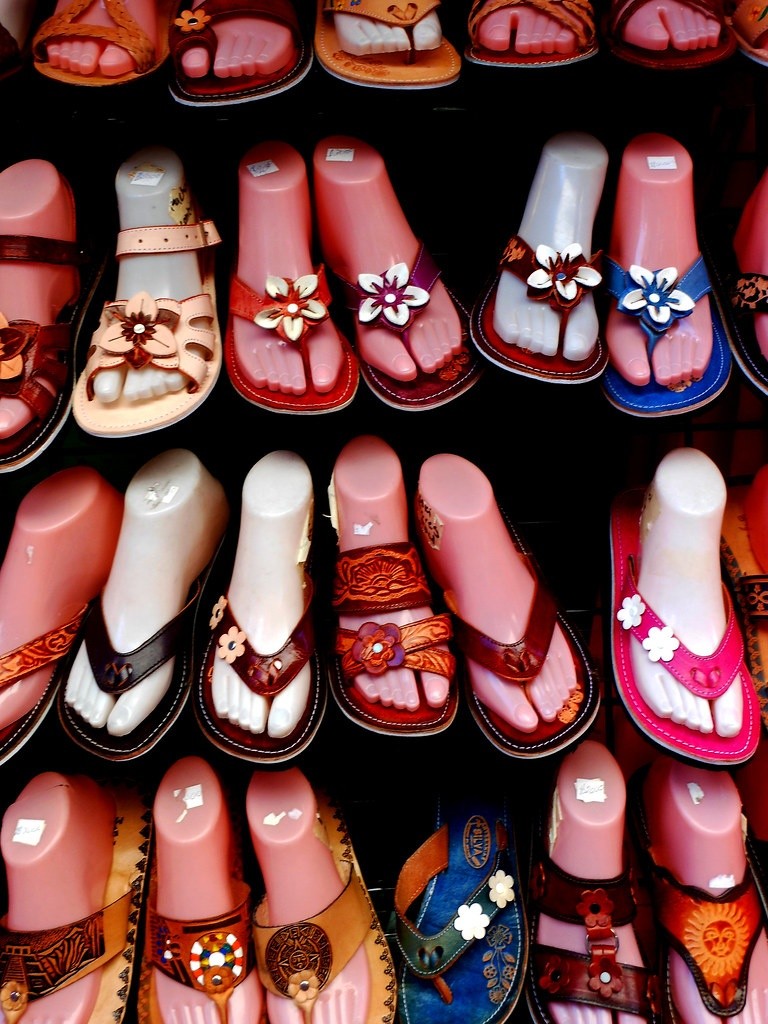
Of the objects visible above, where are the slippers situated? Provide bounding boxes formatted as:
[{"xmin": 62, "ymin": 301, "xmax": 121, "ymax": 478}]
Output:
[
  {"xmin": 0, "ymin": 600, "xmax": 88, "ymax": 766},
  {"xmin": 629, "ymin": 765, "xmax": 768, "ymax": 1024},
  {"xmin": 54, "ymin": 530, "xmax": 225, "ymax": 762},
  {"xmin": 0, "ymin": 778, "xmax": 152, "ymax": 1024},
  {"xmin": 324, "ymin": 538, "xmax": 461, "ymax": 738},
  {"xmin": 250, "ymin": 789, "xmax": 399, "ymax": 1024},
  {"xmin": 523, "ymin": 821, "xmax": 662, "ymax": 1024},
  {"xmin": 607, "ymin": 484, "xmax": 761, "ymax": 767},
  {"xmin": 384, "ymin": 771, "xmax": 531, "ymax": 1024},
  {"xmin": 192, "ymin": 571, "xmax": 330, "ymax": 767},
  {"xmin": 0, "ymin": 185, "xmax": 116, "ymax": 474},
  {"xmin": 437, "ymin": 500, "xmax": 602, "ymax": 759},
  {"xmin": 136, "ymin": 811, "xmax": 268, "ymax": 1024},
  {"xmin": 719, "ymin": 477, "xmax": 768, "ymax": 736},
  {"xmin": 25, "ymin": 0, "xmax": 768, "ymax": 441}
]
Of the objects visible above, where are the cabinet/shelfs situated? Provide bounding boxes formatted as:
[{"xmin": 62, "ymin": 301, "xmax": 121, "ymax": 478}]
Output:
[{"xmin": 0, "ymin": 0, "xmax": 768, "ymax": 1024}]
